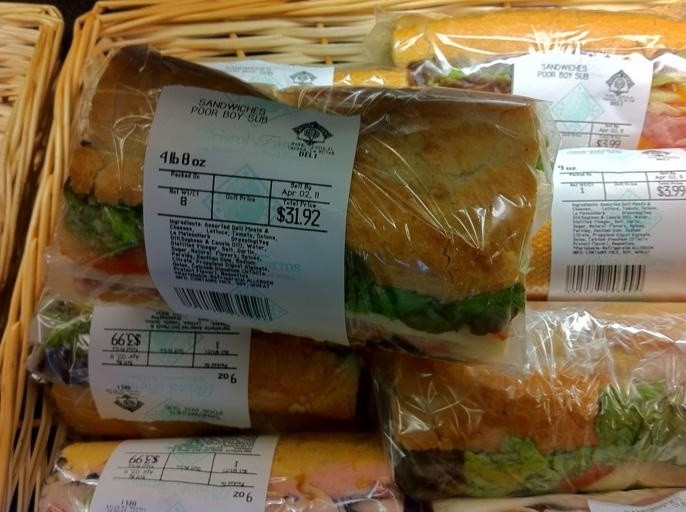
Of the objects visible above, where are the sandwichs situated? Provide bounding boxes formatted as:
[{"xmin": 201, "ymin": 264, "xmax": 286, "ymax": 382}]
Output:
[
  {"xmin": 523, "ymin": 163, "xmax": 686, "ymax": 305},
  {"xmin": 35, "ymin": 425, "xmax": 403, "ymax": 510},
  {"xmin": 190, "ymin": 57, "xmax": 411, "ymax": 88},
  {"xmin": 382, "ymin": 299, "xmax": 686, "ymax": 498},
  {"xmin": 57, "ymin": 43, "xmax": 538, "ymax": 363},
  {"xmin": 35, "ymin": 283, "xmax": 362, "ymax": 440},
  {"xmin": 391, "ymin": 6, "xmax": 686, "ymax": 67}
]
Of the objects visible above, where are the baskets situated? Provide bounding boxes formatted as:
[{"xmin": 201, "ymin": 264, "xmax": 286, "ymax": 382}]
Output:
[
  {"xmin": 1, "ymin": 2, "xmax": 599, "ymax": 511},
  {"xmin": 0, "ymin": 3, "xmax": 65, "ymax": 300}
]
